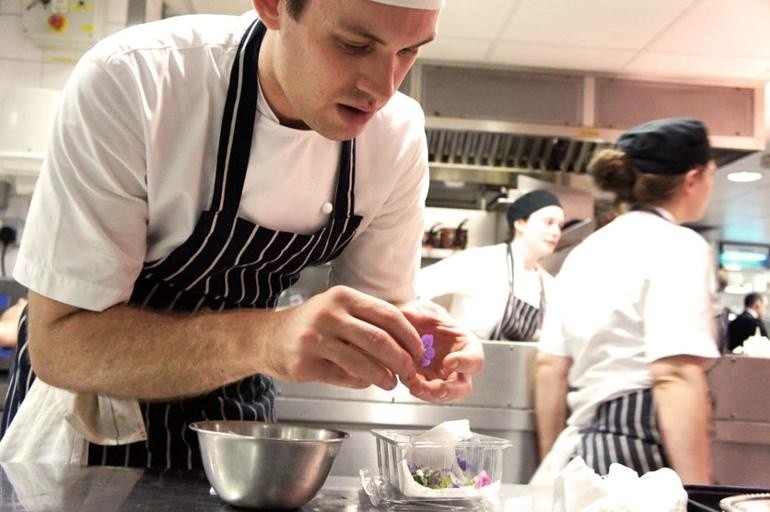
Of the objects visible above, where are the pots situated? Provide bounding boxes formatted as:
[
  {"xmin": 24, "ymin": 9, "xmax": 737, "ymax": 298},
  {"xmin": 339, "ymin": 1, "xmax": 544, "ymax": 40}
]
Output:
[{"xmin": 422, "ymin": 217, "xmax": 473, "ymax": 249}]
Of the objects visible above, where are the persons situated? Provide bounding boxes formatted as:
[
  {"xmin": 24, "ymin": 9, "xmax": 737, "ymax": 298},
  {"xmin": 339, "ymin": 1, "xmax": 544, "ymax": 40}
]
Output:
[
  {"xmin": 727, "ymin": 292, "xmax": 770, "ymax": 351},
  {"xmin": 1, "ymin": 0, "xmax": 483, "ymax": 487},
  {"xmin": 1, "ymin": 227, "xmax": 20, "ymax": 277},
  {"xmin": 414, "ymin": 191, "xmax": 567, "ymax": 342},
  {"xmin": 532, "ymin": 116, "xmax": 725, "ymax": 488}
]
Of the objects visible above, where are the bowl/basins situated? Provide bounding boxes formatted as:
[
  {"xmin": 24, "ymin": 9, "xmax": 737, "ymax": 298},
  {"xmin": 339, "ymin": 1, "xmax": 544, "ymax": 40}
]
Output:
[{"xmin": 187, "ymin": 419, "xmax": 353, "ymax": 511}]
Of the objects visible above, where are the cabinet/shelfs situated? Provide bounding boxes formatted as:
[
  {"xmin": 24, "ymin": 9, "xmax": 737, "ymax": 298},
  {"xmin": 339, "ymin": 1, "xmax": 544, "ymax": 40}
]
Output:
[
  {"xmin": 420, "ymin": 175, "xmax": 598, "ymax": 278},
  {"xmin": 708, "ymin": 234, "xmax": 770, "ymax": 339}
]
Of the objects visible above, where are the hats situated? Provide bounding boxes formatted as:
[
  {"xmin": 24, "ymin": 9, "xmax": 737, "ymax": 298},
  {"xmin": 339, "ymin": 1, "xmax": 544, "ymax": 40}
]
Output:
[
  {"xmin": 617, "ymin": 117, "xmax": 711, "ymax": 177},
  {"xmin": 507, "ymin": 191, "xmax": 561, "ymax": 223}
]
